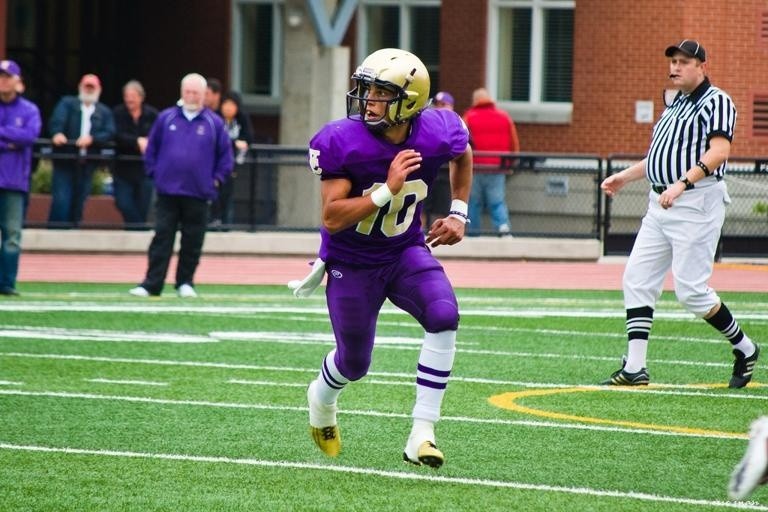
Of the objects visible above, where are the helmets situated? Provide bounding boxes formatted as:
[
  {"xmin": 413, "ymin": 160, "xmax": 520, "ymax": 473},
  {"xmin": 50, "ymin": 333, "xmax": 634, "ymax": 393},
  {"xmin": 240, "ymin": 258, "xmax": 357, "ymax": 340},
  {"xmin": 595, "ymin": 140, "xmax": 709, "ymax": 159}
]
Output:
[{"xmin": 345, "ymin": 47, "xmax": 431, "ymax": 128}]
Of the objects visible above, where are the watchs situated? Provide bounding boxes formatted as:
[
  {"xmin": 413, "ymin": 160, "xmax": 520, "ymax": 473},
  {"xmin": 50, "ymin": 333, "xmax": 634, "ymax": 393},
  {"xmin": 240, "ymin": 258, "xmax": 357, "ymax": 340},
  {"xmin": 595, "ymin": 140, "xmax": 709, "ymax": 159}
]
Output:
[{"xmin": 680, "ymin": 176, "xmax": 691, "ymax": 190}]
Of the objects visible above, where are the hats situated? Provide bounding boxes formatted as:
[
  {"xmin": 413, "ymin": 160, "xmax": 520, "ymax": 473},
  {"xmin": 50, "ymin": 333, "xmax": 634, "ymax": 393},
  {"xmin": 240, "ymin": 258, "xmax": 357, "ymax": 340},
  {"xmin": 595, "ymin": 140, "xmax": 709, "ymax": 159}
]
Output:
[
  {"xmin": 80, "ymin": 74, "xmax": 100, "ymax": 88},
  {"xmin": 665, "ymin": 39, "xmax": 705, "ymax": 61},
  {"xmin": 0, "ymin": 60, "xmax": 21, "ymax": 76},
  {"xmin": 433, "ymin": 91, "xmax": 454, "ymax": 106}
]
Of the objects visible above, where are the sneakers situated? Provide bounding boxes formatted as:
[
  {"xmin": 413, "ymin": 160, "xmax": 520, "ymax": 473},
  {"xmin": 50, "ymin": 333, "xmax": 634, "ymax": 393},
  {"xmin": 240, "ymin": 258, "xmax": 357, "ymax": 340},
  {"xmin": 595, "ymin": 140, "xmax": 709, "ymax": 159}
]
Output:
[
  {"xmin": 600, "ymin": 354, "xmax": 651, "ymax": 386},
  {"xmin": 729, "ymin": 341, "xmax": 759, "ymax": 389},
  {"xmin": 307, "ymin": 379, "xmax": 341, "ymax": 457},
  {"xmin": 178, "ymin": 283, "xmax": 196, "ymax": 297},
  {"xmin": 129, "ymin": 287, "xmax": 148, "ymax": 297},
  {"xmin": 403, "ymin": 440, "xmax": 444, "ymax": 467}
]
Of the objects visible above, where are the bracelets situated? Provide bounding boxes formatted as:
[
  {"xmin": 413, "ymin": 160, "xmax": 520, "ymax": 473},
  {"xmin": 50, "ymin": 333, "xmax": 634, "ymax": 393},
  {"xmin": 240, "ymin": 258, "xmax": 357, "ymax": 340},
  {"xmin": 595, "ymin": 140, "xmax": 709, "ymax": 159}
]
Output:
[
  {"xmin": 697, "ymin": 160, "xmax": 710, "ymax": 176},
  {"xmin": 370, "ymin": 183, "xmax": 393, "ymax": 208},
  {"xmin": 450, "ymin": 198, "xmax": 470, "ymax": 224}
]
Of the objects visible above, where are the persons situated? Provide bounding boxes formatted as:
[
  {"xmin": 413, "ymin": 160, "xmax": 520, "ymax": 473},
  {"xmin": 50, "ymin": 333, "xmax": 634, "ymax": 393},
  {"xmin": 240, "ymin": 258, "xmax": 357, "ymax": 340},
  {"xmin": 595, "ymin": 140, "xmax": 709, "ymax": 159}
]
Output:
[
  {"xmin": 113, "ymin": 80, "xmax": 160, "ymax": 230},
  {"xmin": 307, "ymin": 48, "xmax": 473, "ymax": 468},
  {"xmin": 462, "ymin": 86, "xmax": 520, "ymax": 236},
  {"xmin": 1, "ymin": 60, "xmax": 42, "ymax": 296},
  {"xmin": 601, "ymin": 40, "xmax": 759, "ymax": 389},
  {"xmin": 433, "ymin": 92, "xmax": 456, "ymax": 109},
  {"xmin": 129, "ymin": 73, "xmax": 236, "ymax": 296},
  {"xmin": 219, "ymin": 93, "xmax": 248, "ymax": 150},
  {"xmin": 204, "ymin": 80, "xmax": 252, "ymax": 229},
  {"xmin": 46, "ymin": 74, "xmax": 114, "ymax": 228}
]
